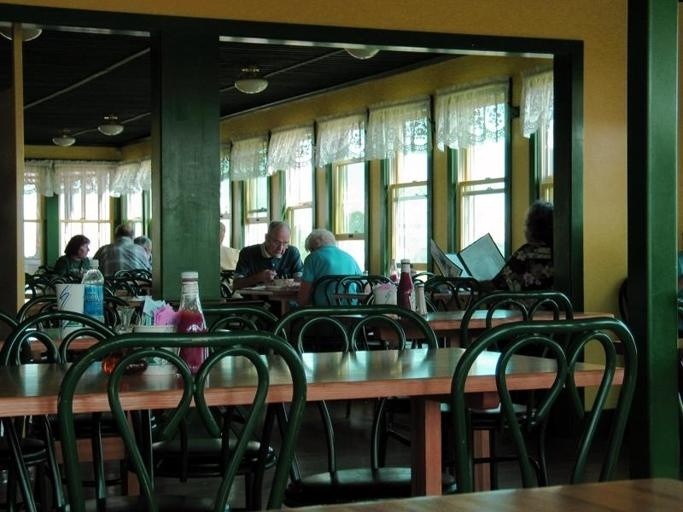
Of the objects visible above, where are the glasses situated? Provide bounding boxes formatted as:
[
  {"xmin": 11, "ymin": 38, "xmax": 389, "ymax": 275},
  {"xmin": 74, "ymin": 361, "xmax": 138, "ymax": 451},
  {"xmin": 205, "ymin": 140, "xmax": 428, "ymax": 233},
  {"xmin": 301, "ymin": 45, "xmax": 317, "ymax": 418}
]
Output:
[{"xmin": 269, "ymin": 236, "xmax": 289, "ymax": 249}]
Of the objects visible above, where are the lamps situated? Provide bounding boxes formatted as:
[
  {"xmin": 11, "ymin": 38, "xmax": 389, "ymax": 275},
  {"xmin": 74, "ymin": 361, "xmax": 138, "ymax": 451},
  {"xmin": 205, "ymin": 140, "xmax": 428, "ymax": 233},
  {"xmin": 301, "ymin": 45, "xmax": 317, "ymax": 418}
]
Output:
[
  {"xmin": 235, "ymin": 66, "xmax": 268, "ymax": 94},
  {"xmin": 0, "ymin": 27, "xmax": 42, "ymax": 41},
  {"xmin": 345, "ymin": 48, "xmax": 380, "ymax": 60},
  {"xmin": 53, "ymin": 127, "xmax": 76, "ymax": 146},
  {"xmin": 98, "ymin": 114, "xmax": 124, "ymax": 135}
]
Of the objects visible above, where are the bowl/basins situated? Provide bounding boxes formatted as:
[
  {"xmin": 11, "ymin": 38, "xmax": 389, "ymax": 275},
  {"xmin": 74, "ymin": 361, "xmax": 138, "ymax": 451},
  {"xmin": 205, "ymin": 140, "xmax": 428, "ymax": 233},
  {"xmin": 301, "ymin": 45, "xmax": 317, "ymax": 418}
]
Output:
[{"xmin": 272, "ymin": 278, "xmax": 295, "ymax": 286}]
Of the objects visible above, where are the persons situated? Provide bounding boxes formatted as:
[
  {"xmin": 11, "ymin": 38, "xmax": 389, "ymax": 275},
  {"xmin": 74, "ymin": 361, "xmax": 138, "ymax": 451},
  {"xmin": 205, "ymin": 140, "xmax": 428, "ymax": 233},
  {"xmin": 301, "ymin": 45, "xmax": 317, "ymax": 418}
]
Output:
[
  {"xmin": 234, "ymin": 221, "xmax": 304, "ymax": 332},
  {"xmin": 288, "ymin": 229, "xmax": 364, "ymax": 352},
  {"xmin": 220, "ymin": 222, "xmax": 245, "ymax": 299},
  {"xmin": 133, "ymin": 235, "xmax": 152, "ymax": 267},
  {"xmin": 468, "ymin": 200, "xmax": 554, "ymax": 310},
  {"xmin": 677, "ymin": 250, "xmax": 683, "ymax": 338},
  {"xmin": 94, "ymin": 224, "xmax": 151, "ymax": 279},
  {"xmin": 53, "ymin": 235, "xmax": 92, "ymax": 276}
]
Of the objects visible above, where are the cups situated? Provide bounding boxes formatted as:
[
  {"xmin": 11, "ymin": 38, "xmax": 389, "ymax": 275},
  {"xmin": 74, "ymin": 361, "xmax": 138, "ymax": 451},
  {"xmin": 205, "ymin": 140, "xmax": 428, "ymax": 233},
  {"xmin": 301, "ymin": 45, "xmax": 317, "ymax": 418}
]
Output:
[
  {"xmin": 55, "ymin": 283, "xmax": 85, "ymax": 330},
  {"xmin": 135, "ymin": 324, "xmax": 179, "ymax": 367},
  {"xmin": 373, "ymin": 288, "xmax": 397, "ymax": 318}
]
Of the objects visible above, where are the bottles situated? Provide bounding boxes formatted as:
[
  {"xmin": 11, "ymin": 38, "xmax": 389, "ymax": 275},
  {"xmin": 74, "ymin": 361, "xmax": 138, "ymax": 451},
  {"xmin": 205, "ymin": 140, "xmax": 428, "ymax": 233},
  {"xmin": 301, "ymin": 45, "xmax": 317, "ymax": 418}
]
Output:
[
  {"xmin": 177, "ymin": 270, "xmax": 209, "ymax": 373},
  {"xmin": 415, "ymin": 281, "xmax": 428, "ymax": 316},
  {"xmin": 397, "ymin": 258, "xmax": 416, "ymax": 317},
  {"xmin": 83, "ymin": 259, "xmax": 105, "ymax": 330}
]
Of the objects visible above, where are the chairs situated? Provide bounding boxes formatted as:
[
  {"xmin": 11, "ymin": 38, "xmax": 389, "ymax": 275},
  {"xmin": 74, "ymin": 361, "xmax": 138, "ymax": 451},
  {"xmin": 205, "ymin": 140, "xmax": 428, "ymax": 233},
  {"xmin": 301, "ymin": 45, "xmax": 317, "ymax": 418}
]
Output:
[
  {"xmin": 462, "ymin": 292, "xmax": 575, "ymax": 490},
  {"xmin": 313, "ymin": 274, "xmax": 516, "ymax": 308},
  {"xmin": 266, "ymin": 306, "xmax": 450, "ymax": 511},
  {"xmin": 451, "ymin": 317, "xmax": 637, "ymax": 492}
]
